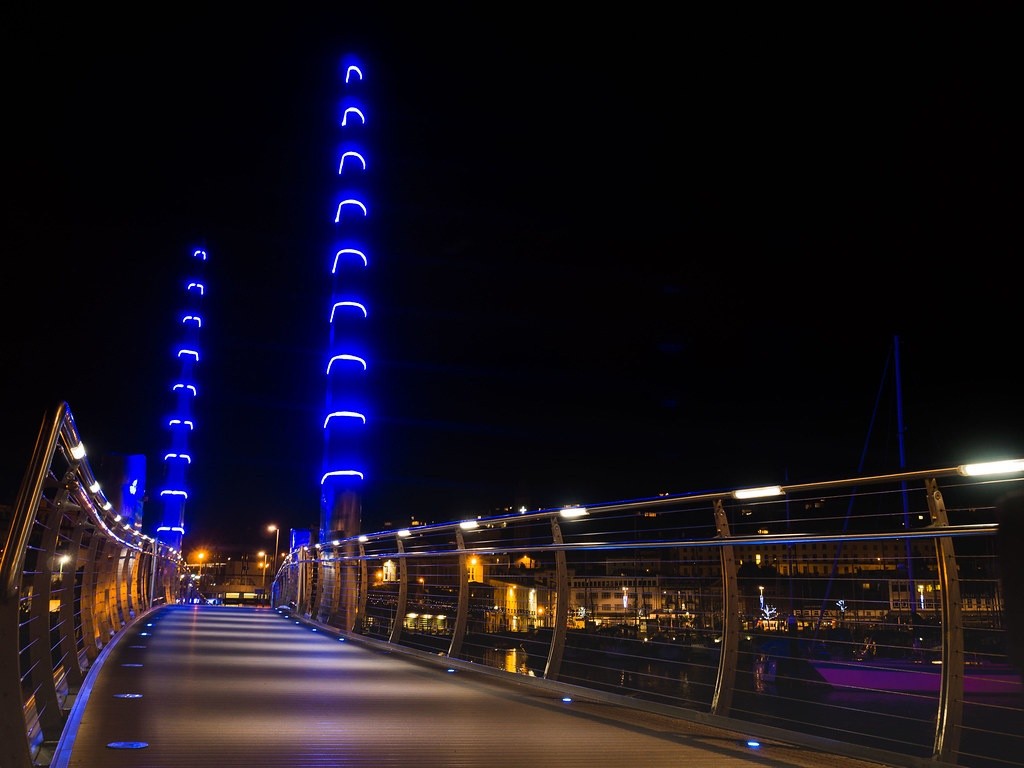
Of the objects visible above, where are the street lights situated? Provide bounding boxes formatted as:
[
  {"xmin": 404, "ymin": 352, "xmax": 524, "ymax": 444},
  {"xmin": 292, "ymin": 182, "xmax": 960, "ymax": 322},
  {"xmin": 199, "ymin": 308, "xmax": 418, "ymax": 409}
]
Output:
[
  {"xmin": 258, "ymin": 551, "xmax": 267, "ymax": 606},
  {"xmin": 622, "ymin": 587, "xmax": 628, "ymax": 624},
  {"xmin": 198, "ymin": 553, "xmax": 204, "ymax": 589},
  {"xmin": 268, "ymin": 525, "xmax": 280, "ymax": 580}
]
